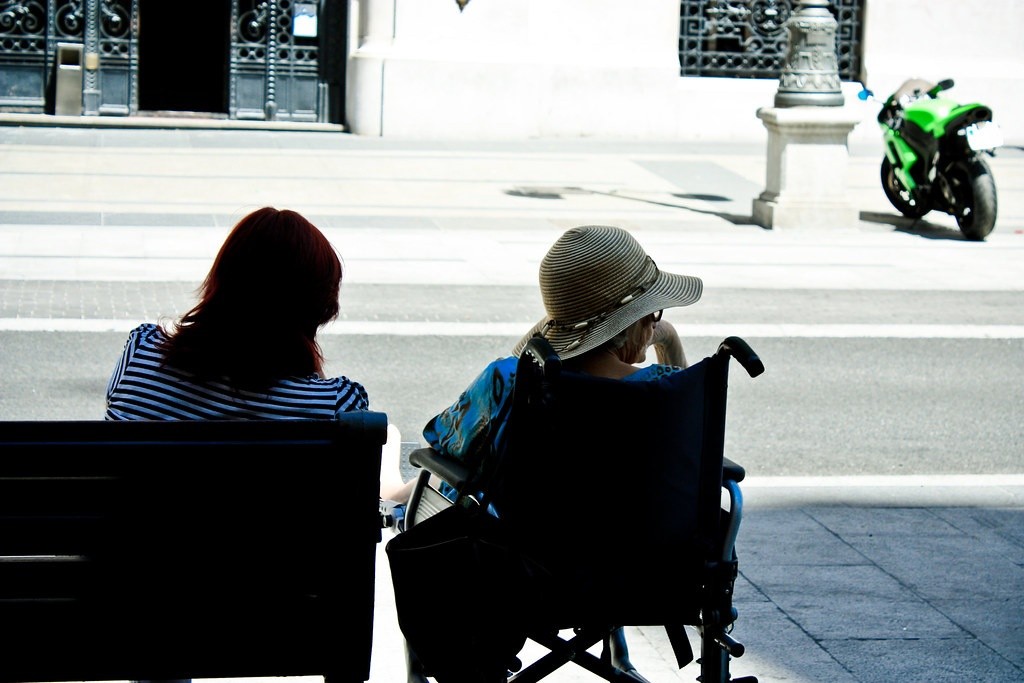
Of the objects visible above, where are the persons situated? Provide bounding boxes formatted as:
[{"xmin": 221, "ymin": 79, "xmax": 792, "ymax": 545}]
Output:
[
  {"xmin": 104, "ymin": 206, "xmax": 370, "ymax": 422},
  {"xmin": 384, "ymin": 225, "xmax": 703, "ymax": 534}
]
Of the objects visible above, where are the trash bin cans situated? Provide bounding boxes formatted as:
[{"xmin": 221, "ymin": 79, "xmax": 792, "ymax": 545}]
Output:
[{"xmin": 54, "ymin": 42, "xmax": 84, "ymax": 117}]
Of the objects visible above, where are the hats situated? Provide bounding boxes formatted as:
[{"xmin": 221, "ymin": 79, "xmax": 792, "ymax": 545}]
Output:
[{"xmin": 511, "ymin": 225, "xmax": 703, "ymax": 361}]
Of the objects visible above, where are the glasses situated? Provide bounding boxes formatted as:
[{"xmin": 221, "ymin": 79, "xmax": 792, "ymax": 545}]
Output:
[{"xmin": 651, "ymin": 309, "xmax": 664, "ymax": 322}]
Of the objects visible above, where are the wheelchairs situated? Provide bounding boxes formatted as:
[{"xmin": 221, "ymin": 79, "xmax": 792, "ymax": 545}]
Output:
[{"xmin": 383, "ymin": 335, "xmax": 766, "ymax": 683}]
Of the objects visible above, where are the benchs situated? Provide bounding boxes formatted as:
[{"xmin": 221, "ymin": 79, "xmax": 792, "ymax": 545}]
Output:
[{"xmin": 1, "ymin": 410, "xmax": 387, "ymax": 683}]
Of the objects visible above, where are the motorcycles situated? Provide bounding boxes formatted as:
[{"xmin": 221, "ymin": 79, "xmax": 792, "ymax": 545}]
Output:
[{"xmin": 859, "ymin": 78, "xmax": 998, "ymax": 241}]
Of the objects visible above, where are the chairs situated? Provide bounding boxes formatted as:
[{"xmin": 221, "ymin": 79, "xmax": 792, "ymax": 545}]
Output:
[{"xmin": 383, "ymin": 336, "xmax": 766, "ymax": 683}]
